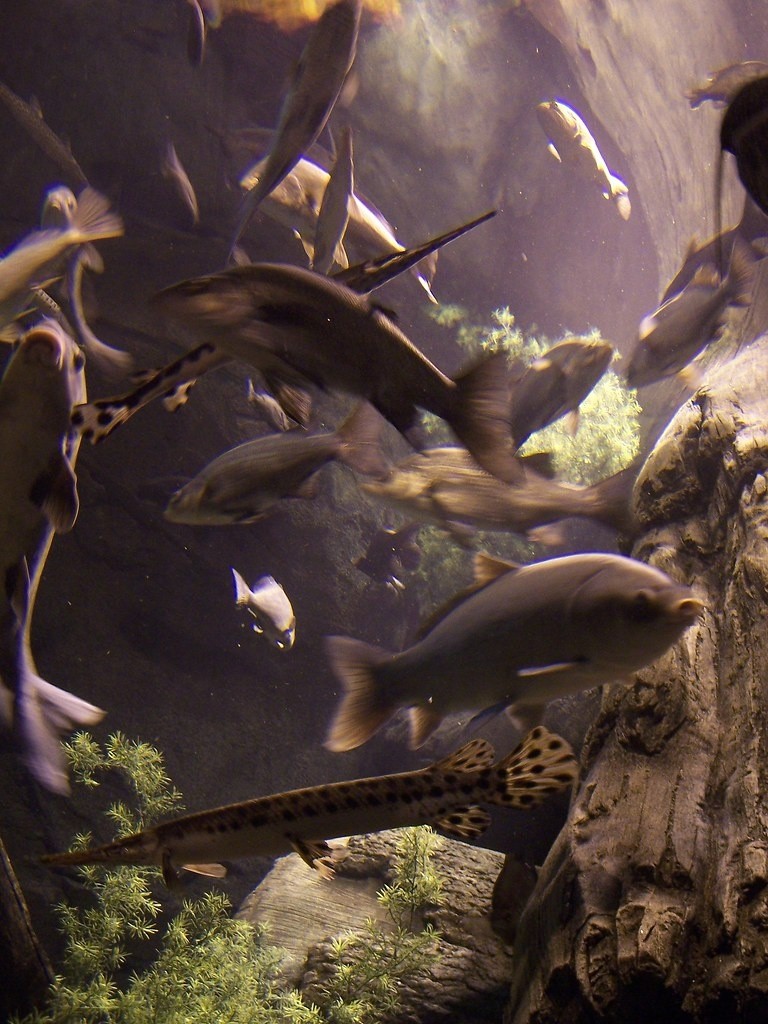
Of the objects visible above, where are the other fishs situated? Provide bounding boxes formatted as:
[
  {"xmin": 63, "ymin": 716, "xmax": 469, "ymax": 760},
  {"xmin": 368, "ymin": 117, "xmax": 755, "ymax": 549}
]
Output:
[{"xmin": 0, "ymin": 0, "xmax": 768, "ymax": 881}]
